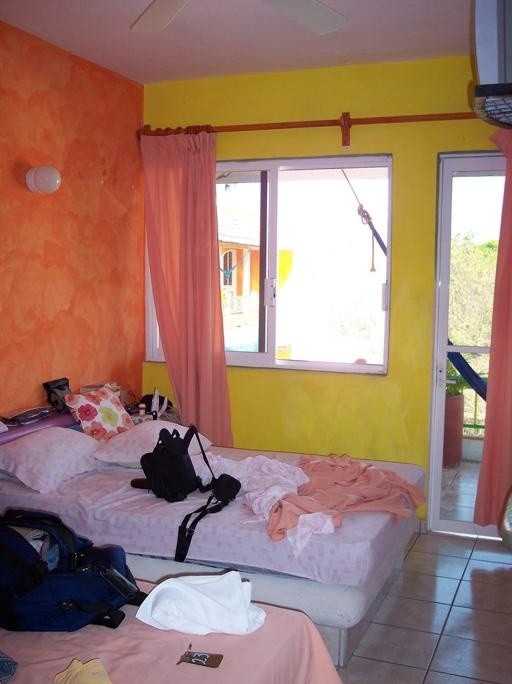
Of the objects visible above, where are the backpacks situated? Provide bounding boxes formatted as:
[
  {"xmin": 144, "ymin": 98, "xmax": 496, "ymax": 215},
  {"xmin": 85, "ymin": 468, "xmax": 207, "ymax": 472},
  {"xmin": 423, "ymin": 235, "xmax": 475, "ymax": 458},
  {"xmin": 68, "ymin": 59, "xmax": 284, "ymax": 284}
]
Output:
[{"xmin": 130, "ymin": 423, "xmax": 215, "ymax": 502}]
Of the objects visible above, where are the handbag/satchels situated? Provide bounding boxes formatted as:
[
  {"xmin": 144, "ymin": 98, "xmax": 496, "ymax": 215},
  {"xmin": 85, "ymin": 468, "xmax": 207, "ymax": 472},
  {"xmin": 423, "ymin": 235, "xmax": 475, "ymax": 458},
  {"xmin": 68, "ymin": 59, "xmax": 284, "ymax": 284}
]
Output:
[
  {"xmin": 175, "ymin": 473, "xmax": 241, "ymax": 562},
  {"xmin": 43, "ymin": 378, "xmax": 72, "ymax": 412}
]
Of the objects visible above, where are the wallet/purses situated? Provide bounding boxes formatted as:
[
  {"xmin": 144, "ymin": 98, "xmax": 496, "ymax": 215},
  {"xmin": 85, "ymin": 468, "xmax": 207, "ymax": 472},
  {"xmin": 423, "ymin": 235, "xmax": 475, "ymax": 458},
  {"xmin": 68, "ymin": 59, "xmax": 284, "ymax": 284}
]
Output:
[{"xmin": 131, "ymin": 478, "xmax": 153, "ymax": 489}]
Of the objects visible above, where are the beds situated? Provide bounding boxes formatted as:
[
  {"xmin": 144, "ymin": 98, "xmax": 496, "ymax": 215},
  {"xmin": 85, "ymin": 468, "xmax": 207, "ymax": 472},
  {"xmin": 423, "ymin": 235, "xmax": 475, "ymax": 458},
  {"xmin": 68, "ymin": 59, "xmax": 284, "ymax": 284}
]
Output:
[
  {"xmin": 0, "ymin": 408, "xmax": 425, "ymax": 668},
  {"xmin": 0, "ymin": 579, "xmax": 344, "ymax": 684},
  {"xmin": 358, "ymin": 203, "xmax": 489, "ymax": 402}
]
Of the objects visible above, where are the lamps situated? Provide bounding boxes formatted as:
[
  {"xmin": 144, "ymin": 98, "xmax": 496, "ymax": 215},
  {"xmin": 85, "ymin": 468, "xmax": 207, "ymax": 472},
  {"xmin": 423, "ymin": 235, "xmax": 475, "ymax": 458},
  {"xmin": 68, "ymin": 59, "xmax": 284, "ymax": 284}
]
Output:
[{"xmin": 25, "ymin": 166, "xmax": 61, "ymax": 194}]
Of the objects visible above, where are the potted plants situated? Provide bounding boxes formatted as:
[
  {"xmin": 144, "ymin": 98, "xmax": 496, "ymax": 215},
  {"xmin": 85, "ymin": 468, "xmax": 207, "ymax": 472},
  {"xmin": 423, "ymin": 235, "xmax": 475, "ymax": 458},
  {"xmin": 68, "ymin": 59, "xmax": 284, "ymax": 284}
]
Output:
[{"xmin": 442, "ymin": 359, "xmax": 472, "ymax": 467}]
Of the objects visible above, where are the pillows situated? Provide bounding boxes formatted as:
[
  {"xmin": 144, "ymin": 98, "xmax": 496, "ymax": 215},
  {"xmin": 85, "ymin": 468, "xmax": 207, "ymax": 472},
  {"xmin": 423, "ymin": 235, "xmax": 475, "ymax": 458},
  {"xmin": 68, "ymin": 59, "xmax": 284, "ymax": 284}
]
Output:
[
  {"xmin": 0, "ymin": 426, "xmax": 111, "ymax": 495},
  {"xmin": 64, "ymin": 382, "xmax": 136, "ymax": 446},
  {"xmin": 90, "ymin": 420, "xmax": 213, "ymax": 469}
]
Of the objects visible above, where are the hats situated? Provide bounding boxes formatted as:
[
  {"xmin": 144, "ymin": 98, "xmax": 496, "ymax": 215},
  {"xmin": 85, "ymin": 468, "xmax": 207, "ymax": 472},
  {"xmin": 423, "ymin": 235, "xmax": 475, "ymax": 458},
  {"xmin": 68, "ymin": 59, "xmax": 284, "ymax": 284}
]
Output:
[{"xmin": 128, "ymin": 395, "xmax": 173, "ymax": 414}]
